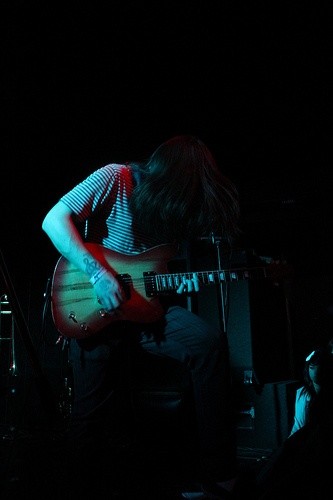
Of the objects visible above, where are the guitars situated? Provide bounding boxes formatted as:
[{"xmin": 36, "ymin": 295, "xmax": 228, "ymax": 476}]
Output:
[{"xmin": 49, "ymin": 242, "xmax": 295, "ymax": 341}]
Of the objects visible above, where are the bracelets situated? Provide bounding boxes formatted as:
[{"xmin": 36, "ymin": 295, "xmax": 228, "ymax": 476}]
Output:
[{"xmin": 88, "ymin": 266, "xmax": 107, "ymax": 284}]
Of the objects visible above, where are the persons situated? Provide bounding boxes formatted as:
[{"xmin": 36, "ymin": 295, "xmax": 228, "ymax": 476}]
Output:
[
  {"xmin": 41, "ymin": 136, "xmax": 239, "ymax": 371},
  {"xmin": 287, "ymin": 350, "xmax": 333, "ymax": 434}
]
